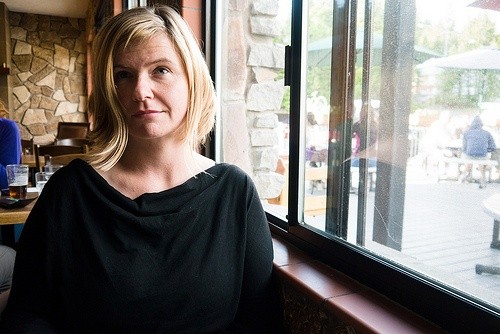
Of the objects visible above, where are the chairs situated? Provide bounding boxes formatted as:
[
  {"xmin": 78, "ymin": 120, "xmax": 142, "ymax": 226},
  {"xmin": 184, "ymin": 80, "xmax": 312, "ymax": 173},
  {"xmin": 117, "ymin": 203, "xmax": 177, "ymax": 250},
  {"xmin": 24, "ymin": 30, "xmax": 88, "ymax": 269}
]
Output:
[{"xmin": 21, "ymin": 122, "xmax": 91, "ymax": 172}]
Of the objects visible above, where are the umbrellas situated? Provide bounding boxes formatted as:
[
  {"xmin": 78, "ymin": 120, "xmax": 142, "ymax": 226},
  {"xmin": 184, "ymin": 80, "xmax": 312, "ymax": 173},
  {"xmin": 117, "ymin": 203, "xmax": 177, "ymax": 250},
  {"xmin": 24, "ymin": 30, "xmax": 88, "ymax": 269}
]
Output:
[
  {"xmin": 308, "ymin": 28, "xmax": 442, "ymax": 70},
  {"xmin": 425, "ymin": 44, "xmax": 500, "ymax": 95}
]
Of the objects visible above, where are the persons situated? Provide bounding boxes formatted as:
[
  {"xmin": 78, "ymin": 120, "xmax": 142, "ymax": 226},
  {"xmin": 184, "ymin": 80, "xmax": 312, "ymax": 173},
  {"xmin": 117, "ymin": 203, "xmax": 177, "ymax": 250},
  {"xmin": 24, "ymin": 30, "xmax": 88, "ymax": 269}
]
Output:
[
  {"xmin": 0, "ymin": 117, "xmax": 21, "ymax": 190},
  {"xmin": 462, "ymin": 115, "xmax": 496, "ymax": 181},
  {"xmin": 0, "ymin": 6, "xmax": 273, "ymax": 334},
  {"xmin": 353, "ymin": 105, "xmax": 379, "ymax": 167}
]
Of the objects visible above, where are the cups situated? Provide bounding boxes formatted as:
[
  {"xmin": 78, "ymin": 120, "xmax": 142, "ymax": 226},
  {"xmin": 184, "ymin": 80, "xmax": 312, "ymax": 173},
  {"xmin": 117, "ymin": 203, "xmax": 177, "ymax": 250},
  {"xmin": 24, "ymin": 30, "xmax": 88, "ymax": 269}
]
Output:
[
  {"xmin": 35, "ymin": 172, "xmax": 54, "ymax": 196},
  {"xmin": 6, "ymin": 165, "xmax": 29, "ymax": 200},
  {"xmin": 44, "ymin": 164, "xmax": 64, "ymax": 173}
]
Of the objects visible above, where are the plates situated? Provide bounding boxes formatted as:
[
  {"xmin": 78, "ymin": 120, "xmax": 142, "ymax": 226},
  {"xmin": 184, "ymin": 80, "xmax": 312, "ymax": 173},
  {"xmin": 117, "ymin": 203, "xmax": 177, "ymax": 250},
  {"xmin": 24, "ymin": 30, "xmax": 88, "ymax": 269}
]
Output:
[{"xmin": 0, "ymin": 192, "xmax": 40, "ymax": 209}]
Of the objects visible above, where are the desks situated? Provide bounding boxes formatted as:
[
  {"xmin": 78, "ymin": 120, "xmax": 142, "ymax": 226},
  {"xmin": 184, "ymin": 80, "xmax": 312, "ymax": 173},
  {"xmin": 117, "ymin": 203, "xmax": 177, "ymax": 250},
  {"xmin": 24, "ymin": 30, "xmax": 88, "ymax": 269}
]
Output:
[
  {"xmin": 22, "ymin": 156, "xmax": 53, "ymax": 187},
  {"xmin": 0, "ymin": 192, "xmax": 39, "ymax": 225}
]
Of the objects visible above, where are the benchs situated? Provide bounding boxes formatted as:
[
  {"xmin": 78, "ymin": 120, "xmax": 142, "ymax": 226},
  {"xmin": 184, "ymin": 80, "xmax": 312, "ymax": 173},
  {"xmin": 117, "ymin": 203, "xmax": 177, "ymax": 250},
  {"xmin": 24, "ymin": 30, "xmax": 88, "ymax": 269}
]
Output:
[{"xmin": 443, "ymin": 158, "xmax": 498, "ymax": 189}]
